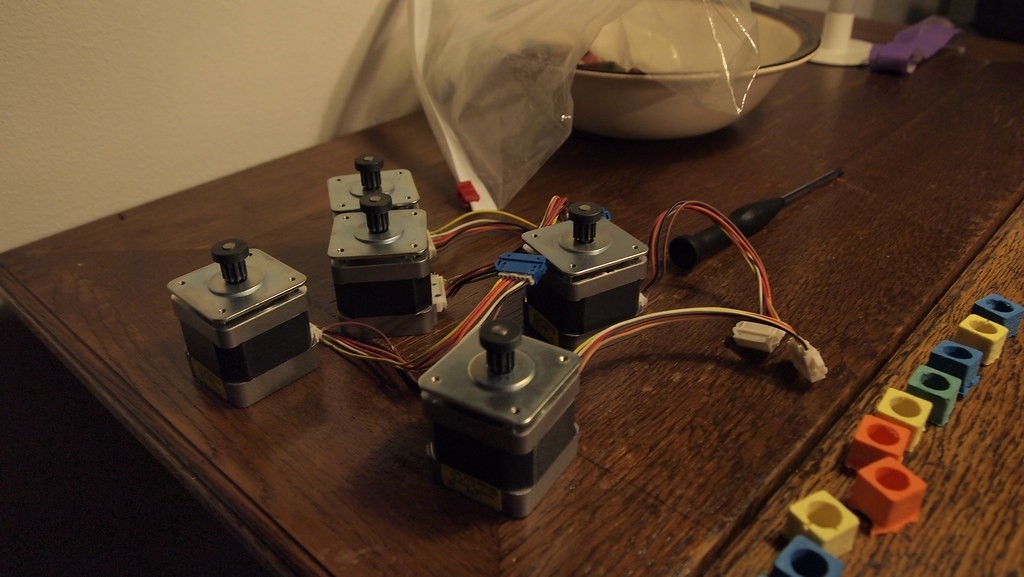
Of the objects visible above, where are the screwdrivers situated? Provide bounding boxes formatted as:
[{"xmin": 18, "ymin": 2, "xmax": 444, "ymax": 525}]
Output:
[{"xmin": 668, "ymin": 167, "xmax": 844, "ymax": 268}]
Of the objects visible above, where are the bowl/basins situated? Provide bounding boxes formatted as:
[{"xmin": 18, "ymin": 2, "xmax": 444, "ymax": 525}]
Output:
[{"xmin": 495, "ymin": 0, "xmax": 821, "ymax": 141}]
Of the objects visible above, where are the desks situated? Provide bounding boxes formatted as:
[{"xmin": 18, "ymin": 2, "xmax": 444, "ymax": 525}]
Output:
[{"xmin": 2, "ymin": 5, "xmax": 1024, "ymax": 575}]
